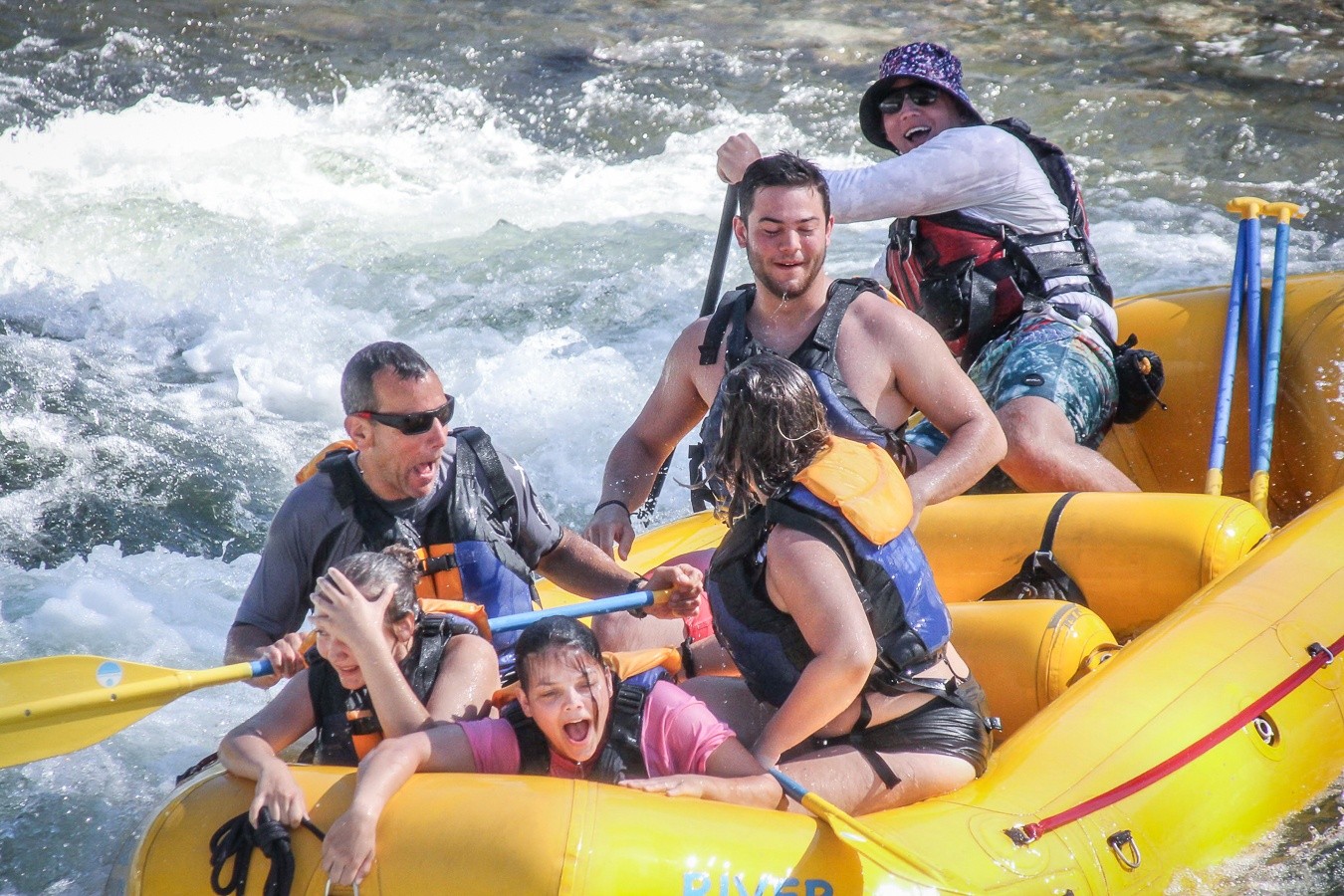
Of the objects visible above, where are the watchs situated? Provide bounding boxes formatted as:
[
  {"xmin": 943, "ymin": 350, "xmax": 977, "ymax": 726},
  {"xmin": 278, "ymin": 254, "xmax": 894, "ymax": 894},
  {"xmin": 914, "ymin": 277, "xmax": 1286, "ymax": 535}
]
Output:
[{"xmin": 625, "ymin": 572, "xmax": 650, "ymax": 619}]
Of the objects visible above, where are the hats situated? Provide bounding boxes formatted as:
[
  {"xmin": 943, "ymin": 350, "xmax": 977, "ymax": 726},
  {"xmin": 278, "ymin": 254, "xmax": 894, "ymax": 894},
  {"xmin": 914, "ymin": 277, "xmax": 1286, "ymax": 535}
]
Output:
[{"xmin": 860, "ymin": 41, "xmax": 985, "ymax": 153}]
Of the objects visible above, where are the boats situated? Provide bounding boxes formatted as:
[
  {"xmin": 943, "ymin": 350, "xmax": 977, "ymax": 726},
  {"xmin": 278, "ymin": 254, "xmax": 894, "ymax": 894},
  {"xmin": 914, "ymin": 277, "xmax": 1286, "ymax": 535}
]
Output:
[{"xmin": 120, "ymin": 267, "xmax": 1344, "ymax": 895}]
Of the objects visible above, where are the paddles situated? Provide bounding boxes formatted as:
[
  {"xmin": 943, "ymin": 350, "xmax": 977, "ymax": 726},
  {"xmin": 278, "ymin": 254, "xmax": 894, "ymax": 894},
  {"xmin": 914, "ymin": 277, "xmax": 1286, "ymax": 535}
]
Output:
[
  {"xmin": 637, "ymin": 182, "xmax": 738, "ymax": 531},
  {"xmin": 1200, "ymin": 195, "xmax": 1311, "ymax": 528},
  {"xmin": 764, "ymin": 763, "xmax": 982, "ymax": 895},
  {"xmin": 6, "ymin": 583, "xmax": 688, "ymax": 771}
]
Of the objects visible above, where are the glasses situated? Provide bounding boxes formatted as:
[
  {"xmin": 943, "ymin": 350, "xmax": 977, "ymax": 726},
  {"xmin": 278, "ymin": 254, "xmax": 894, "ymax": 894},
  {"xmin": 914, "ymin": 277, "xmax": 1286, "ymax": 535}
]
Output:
[
  {"xmin": 875, "ymin": 81, "xmax": 937, "ymax": 115},
  {"xmin": 347, "ymin": 395, "xmax": 454, "ymax": 436}
]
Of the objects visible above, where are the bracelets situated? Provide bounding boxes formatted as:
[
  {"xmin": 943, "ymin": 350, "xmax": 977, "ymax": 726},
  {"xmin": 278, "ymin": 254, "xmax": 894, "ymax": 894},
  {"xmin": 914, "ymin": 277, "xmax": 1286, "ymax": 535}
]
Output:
[{"xmin": 594, "ymin": 500, "xmax": 630, "ymax": 518}]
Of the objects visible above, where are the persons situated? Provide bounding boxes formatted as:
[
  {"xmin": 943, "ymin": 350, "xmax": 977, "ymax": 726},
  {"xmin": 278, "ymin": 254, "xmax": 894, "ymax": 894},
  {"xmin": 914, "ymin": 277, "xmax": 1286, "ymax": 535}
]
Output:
[
  {"xmin": 581, "ymin": 153, "xmax": 1008, "ymax": 652},
  {"xmin": 217, "ymin": 543, "xmax": 502, "ymax": 830},
  {"xmin": 677, "ymin": 353, "xmax": 1002, "ymax": 820},
  {"xmin": 222, "ymin": 340, "xmax": 703, "ymax": 688},
  {"xmin": 716, "ymin": 42, "xmax": 1143, "ymax": 493},
  {"xmin": 320, "ymin": 615, "xmax": 783, "ymax": 887}
]
